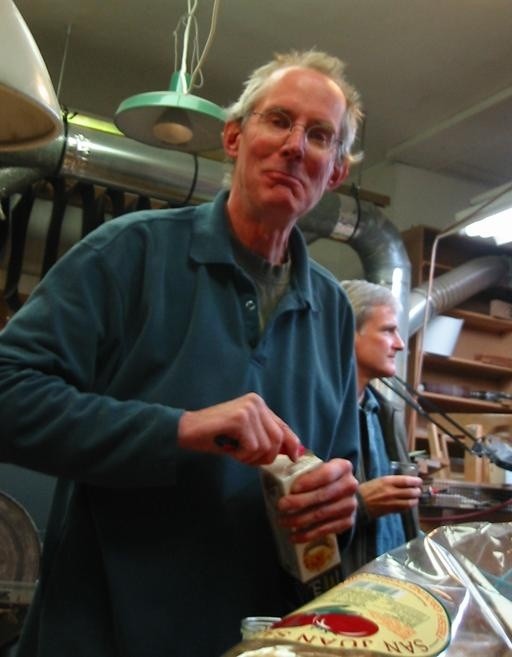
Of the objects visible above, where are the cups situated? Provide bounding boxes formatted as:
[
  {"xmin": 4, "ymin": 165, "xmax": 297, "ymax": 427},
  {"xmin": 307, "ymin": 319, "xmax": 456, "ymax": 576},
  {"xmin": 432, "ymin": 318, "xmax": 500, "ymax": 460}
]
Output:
[{"xmin": 390, "ymin": 461, "xmax": 418, "ymax": 476}]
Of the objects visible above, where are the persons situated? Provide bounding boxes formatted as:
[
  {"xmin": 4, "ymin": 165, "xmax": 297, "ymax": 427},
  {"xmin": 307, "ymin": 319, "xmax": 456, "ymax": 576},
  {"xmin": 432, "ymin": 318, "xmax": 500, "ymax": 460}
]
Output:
[
  {"xmin": 0, "ymin": 49, "xmax": 362, "ymax": 657},
  {"xmin": 336, "ymin": 279, "xmax": 422, "ymax": 557}
]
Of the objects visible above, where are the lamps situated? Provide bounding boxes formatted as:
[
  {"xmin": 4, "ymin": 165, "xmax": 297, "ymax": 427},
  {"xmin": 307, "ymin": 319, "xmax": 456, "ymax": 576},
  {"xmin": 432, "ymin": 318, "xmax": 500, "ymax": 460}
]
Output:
[
  {"xmin": 454, "ymin": 181, "xmax": 512, "ymax": 246},
  {"xmin": 112, "ymin": 1, "xmax": 229, "ymax": 156}
]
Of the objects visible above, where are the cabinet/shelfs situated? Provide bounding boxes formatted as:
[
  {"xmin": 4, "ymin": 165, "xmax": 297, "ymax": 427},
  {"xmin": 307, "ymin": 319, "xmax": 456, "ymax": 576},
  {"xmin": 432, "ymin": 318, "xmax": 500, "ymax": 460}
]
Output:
[{"xmin": 400, "ymin": 224, "xmax": 512, "ymax": 532}]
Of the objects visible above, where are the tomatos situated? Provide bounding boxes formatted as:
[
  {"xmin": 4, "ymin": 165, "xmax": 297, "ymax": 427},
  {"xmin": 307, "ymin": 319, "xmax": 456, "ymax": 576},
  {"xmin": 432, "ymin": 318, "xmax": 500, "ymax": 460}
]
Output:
[
  {"xmin": 311, "ymin": 612, "xmax": 378, "ymax": 639},
  {"xmin": 272, "ymin": 612, "xmax": 315, "ymax": 627}
]
